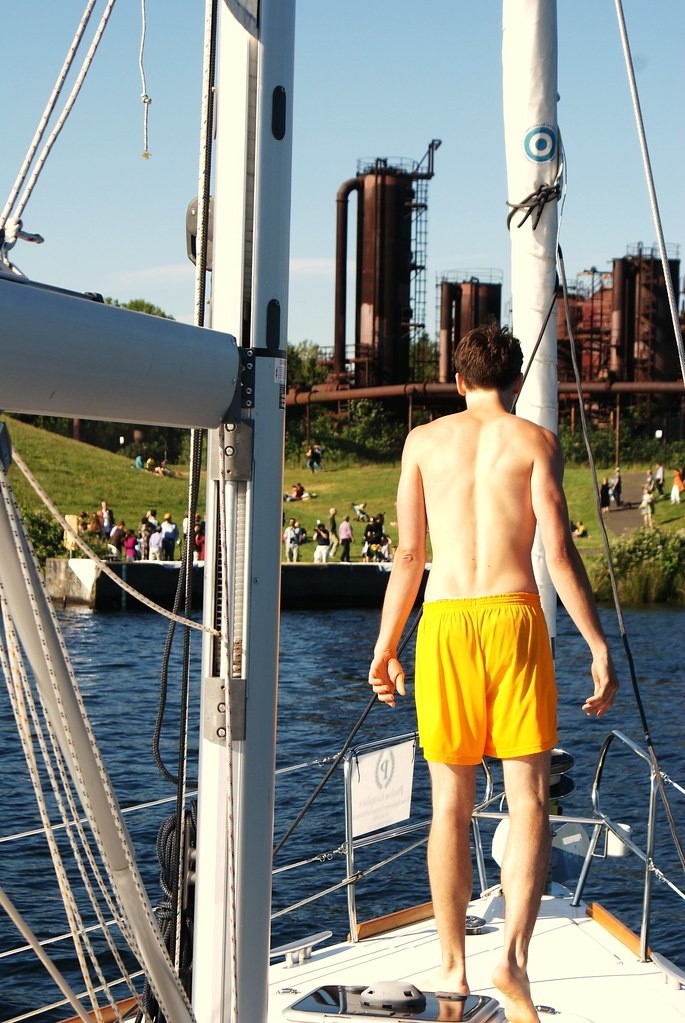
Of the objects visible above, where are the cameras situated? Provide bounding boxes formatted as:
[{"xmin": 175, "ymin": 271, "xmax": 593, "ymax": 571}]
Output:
[{"xmin": 314, "ymin": 529, "xmax": 318, "ymax": 531}]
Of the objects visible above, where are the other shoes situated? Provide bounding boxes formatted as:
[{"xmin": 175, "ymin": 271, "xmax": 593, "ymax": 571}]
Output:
[
  {"xmin": 671, "ymin": 501, "xmax": 675, "ymax": 504},
  {"xmin": 676, "ymin": 502, "xmax": 680, "ymax": 505}
]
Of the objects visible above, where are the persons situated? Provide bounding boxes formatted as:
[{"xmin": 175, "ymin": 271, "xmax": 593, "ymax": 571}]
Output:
[
  {"xmin": 367, "ymin": 321, "xmax": 622, "ymax": 1023},
  {"xmin": 303, "ymin": 440, "xmax": 326, "ymax": 474},
  {"xmin": 599, "ymin": 466, "xmax": 622, "ymax": 515},
  {"xmin": 133, "ymin": 450, "xmax": 170, "ymax": 477},
  {"xmin": 636, "ymin": 463, "xmax": 685, "ymax": 528},
  {"xmin": 570, "ymin": 518, "xmax": 588, "ymax": 538},
  {"xmin": 283, "ymin": 481, "xmax": 310, "ymax": 502},
  {"xmin": 78, "ymin": 502, "xmax": 397, "ymax": 565}
]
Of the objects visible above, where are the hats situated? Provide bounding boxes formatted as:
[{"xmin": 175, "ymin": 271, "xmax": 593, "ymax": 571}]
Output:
[
  {"xmin": 164, "ymin": 513, "xmax": 172, "ymax": 520},
  {"xmin": 616, "ymin": 467, "xmax": 620, "ymax": 470}
]
